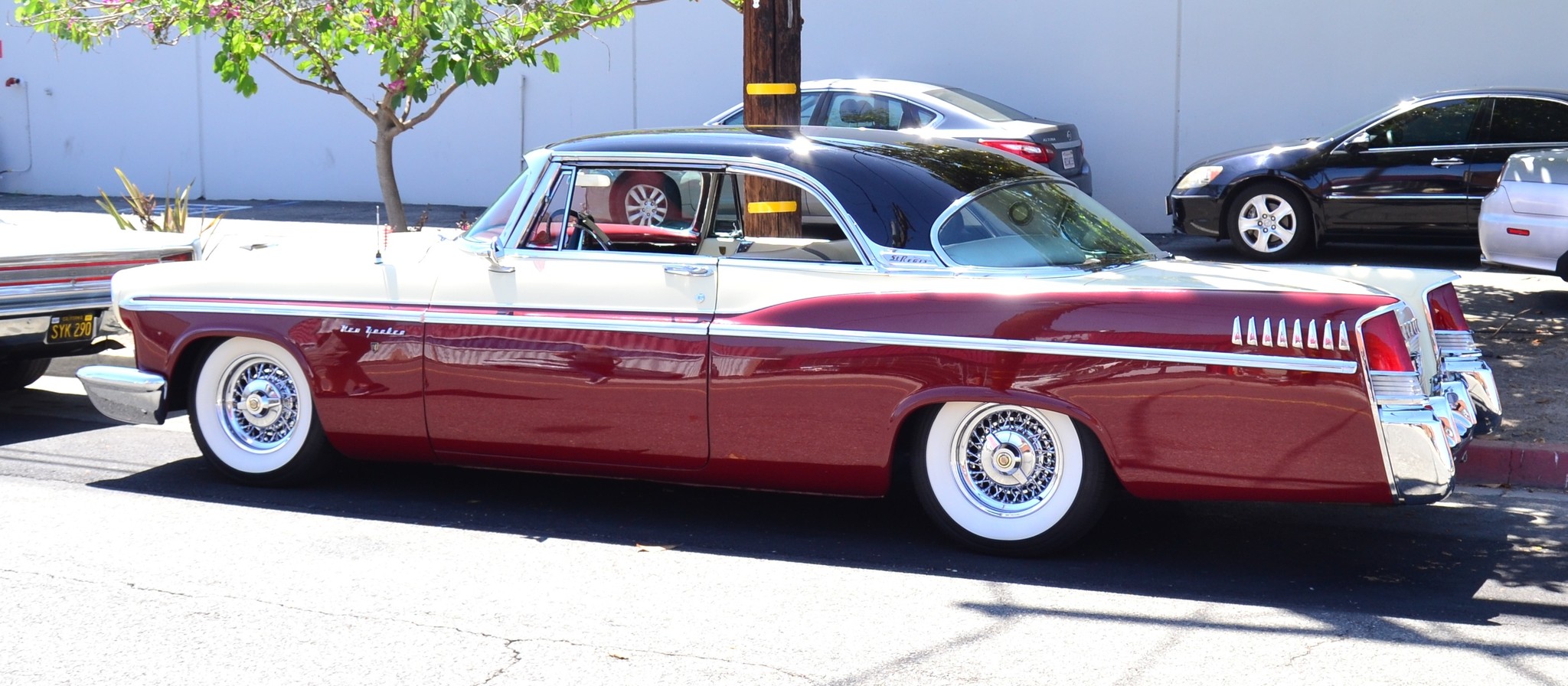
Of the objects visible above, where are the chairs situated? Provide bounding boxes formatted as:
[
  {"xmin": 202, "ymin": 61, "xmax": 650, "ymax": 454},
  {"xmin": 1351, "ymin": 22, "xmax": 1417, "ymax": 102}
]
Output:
[
  {"xmin": 840, "ymin": 99, "xmax": 876, "ymax": 129},
  {"xmin": 731, "ymin": 239, "xmax": 861, "ymax": 262}
]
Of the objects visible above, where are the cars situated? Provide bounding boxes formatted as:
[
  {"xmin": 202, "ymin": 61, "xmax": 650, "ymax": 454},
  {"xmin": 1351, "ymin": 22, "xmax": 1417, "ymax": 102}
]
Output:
[
  {"xmin": 0, "ymin": 210, "xmax": 194, "ymax": 389},
  {"xmin": 520, "ymin": 77, "xmax": 1095, "ymax": 251},
  {"xmin": 1163, "ymin": 87, "xmax": 1567, "ymax": 280},
  {"xmin": 115, "ymin": 125, "xmax": 1501, "ymax": 571}
]
